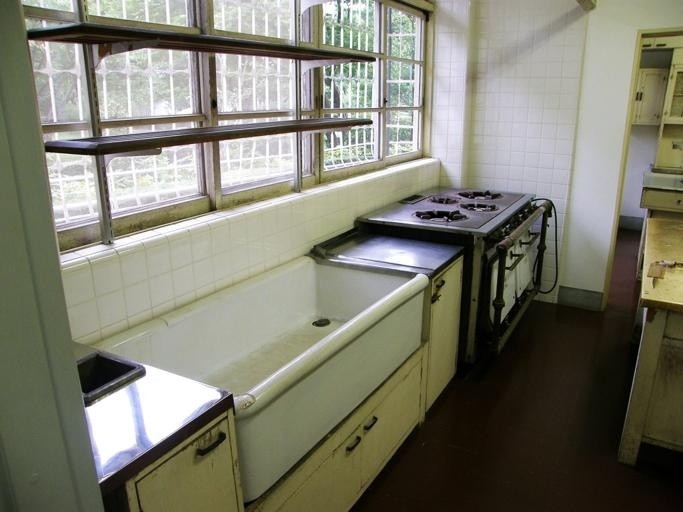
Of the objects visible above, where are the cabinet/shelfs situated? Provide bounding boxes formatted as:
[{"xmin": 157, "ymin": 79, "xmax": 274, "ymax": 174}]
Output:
[
  {"xmin": 640, "ymin": 189, "xmax": 683, "ymax": 214},
  {"xmin": 24, "ymin": 21, "xmax": 377, "ymax": 154},
  {"xmin": 632, "ymin": 68, "xmax": 670, "ymax": 125},
  {"xmin": 652, "ymin": 48, "xmax": 683, "ymax": 175},
  {"xmin": 122, "ymin": 407, "xmax": 245, "ymax": 512},
  {"xmin": 248, "ymin": 341, "xmax": 431, "ymax": 512},
  {"xmin": 641, "ymin": 35, "xmax": 683, "ymax": 50},
  {"xmin": 421, "ymin": 254, "xmax": 464, "ymax": 414}
]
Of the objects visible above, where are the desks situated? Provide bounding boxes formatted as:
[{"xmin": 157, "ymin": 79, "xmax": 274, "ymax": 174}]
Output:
[{"xmin": 616, "ymin": 215, "xmax": 683, "ymax": 468}]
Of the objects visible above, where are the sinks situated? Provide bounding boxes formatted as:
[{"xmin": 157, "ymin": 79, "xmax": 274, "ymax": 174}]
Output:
[{"xmin": 74, "ymin": 348, "xmax": 146, "ymax": 405}]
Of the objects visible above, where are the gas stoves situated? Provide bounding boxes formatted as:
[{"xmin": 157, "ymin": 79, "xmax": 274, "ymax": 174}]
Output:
[{"xmin": 356, "ymin": 184, "xmax": 537, "ymax": 243}]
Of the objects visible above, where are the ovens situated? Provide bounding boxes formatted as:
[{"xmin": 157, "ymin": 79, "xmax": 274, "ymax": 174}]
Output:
[{"xmin": 481, "ymin": 200, "xmax": 552, "ymax": 378}]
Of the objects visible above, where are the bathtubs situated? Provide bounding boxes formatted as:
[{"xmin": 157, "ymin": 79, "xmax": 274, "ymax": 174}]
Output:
[{"xmin": 92, "ymin": 252, "xmax": 430, "ymax": 505}]
